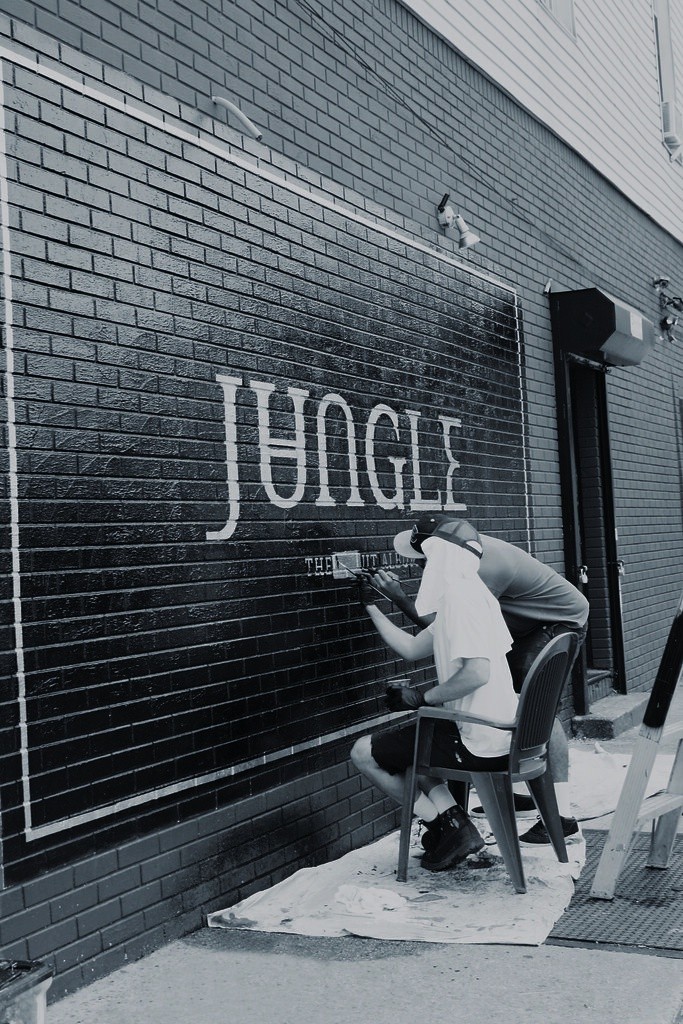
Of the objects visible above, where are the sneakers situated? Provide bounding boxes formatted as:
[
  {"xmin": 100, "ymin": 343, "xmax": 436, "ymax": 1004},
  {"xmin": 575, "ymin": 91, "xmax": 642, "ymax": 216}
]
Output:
[
  {"xmin": 518, "ymin": 814, "xmax": 581, "ymax": 848},
  {"xmin": 470, "ymin": 792, "xmax": 537, "ymax": 817}
]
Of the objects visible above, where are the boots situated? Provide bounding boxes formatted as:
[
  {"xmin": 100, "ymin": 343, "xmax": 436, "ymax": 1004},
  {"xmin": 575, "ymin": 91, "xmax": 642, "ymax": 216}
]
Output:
[{"xmin": 417, "ymin": 804, "xmax": 485, "ymax": 873}]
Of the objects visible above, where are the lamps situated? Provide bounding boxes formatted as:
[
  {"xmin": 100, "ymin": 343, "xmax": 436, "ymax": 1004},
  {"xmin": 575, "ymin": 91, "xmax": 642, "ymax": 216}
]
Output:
[
  {"xmin": 653, "ymin": 275, "xmax": 683, "ymax": 343},
  {"xmin": 437, "ymin": 193, "xmax": 481, "ymax": 250}
]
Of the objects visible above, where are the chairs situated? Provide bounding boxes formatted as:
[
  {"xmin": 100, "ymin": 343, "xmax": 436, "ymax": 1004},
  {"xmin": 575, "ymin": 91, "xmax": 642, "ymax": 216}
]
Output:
[{"xmin": 396, "ymin": 631, "xmax": 580, "ymax": 901}]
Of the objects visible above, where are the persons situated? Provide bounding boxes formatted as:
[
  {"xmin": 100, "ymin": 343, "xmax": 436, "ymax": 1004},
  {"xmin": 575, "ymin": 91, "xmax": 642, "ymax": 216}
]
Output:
[
  {"xmin": 350, "ymin": 519, "xmax": 518, "ymax": 870},
  {"xmin": 369, "ymin": 515, "xmax": 589, "ymax": 848}
]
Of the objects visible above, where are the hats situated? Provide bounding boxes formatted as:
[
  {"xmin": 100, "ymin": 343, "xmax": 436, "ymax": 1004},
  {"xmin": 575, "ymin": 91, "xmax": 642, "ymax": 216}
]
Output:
[
  {"xmin": 418, "ymin": 518, "xmax": 483, "ymax": 559},
  {"xmin": 394, "ymin": 515, "xmax": 451, "ymax": 558}
]
